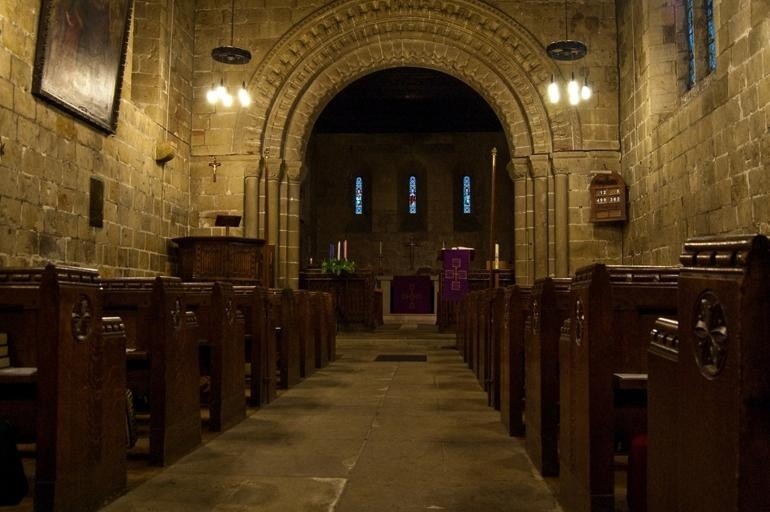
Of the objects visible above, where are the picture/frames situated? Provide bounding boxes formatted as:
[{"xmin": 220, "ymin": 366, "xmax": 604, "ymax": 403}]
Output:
[{"xmin": 31, "ymin": 1, "xmax": 133, "ymax": 136}]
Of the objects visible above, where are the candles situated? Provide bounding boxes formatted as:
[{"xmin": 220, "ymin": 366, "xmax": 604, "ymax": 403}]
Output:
[{"xmin": 337, "ymin": 240, "xmax": 349, "ymax": 262}]
